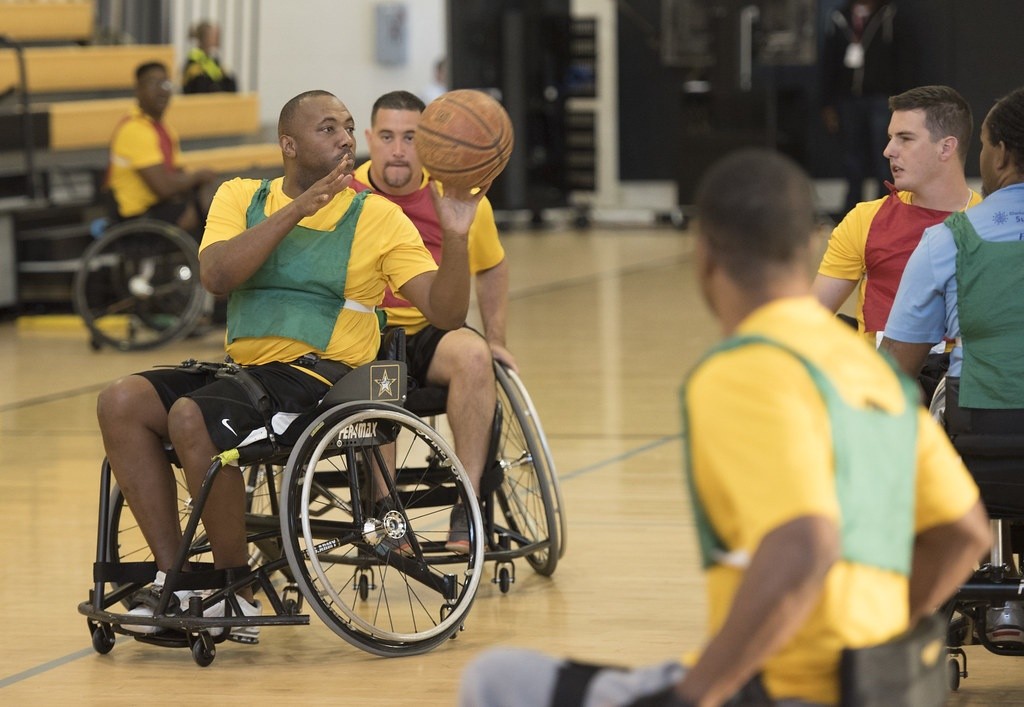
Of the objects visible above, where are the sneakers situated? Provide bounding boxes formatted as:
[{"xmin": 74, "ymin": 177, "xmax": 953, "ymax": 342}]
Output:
[
  {"xmin": 375, "ymin": 497, "xmax": 424, "ymax": 558},
  {"xmin": 985, "ymin": 599, "xmax": 1024, "ymax": 644},
  {"xmin": 444, "ymin": 503, "xmax": 492, "ymax": 554},
  {"xmin": 119, "ymin": 569, "xmax": 263, "ymax": 646}
]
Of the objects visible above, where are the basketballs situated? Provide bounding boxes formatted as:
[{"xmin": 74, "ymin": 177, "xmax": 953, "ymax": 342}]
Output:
[{"xmin": 410, "ymin": 86, "xmax": 518, "ymax": 195}]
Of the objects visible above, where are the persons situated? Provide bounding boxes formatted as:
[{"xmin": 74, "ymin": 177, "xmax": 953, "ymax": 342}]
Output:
[
  {"xmin": 812, "ymin": 88, "xmax": 981, "ymax": 416},
  {"xmin": 96, "ymin": 89, "xmax": 493, "ymax": 646},
  {"xmin": 519, "ymin": 0, "xmax": 906, "ymax": 232},
  {"xmin": 345, "ymin": 91, "xmax": 521, "ymax": 556},
  {"xmin": 456, "ymin": 146, "xmax": 997, "ymax": 706},
  {"xmin": 875, "ymin": 88, "xmax": 1023, "ymax": 646},
  {"xmin": 181, "ymin": 21, "xmax": 239, "ymax": 94},
  {"xmin": 103, "ymin": 62, "xmax": 229, "ymax": 329}
]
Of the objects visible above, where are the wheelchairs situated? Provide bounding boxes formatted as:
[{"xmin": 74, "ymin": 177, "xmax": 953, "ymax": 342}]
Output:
[
  {"xmin": 77, "ymin": 327, "xmax": 484, "ymax": 665},
  {"xmin": 74, "ymin": 217, "xmax": 226, "ymax": 351},
  {"xmin": 941, "ymin": 378, "xmax": 1024, "ymax": 687},
  {"xmin": 279, "ymin": 358, "xmax": 566, "ymax": 596}
]
